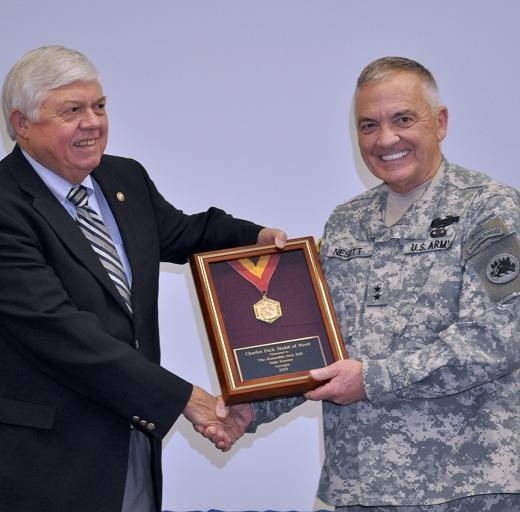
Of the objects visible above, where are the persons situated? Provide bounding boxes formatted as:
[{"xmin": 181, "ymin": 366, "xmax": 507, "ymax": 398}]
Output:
[
  {"xmin": 0, "ymin": 44, "xmax": 288, "ymax": 512},
  {"xmin": 188, "ymin": 55, "xmax": 518, "ymax": 509}
]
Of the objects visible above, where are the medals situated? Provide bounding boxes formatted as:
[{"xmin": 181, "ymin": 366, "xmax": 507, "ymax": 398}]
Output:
[{"xmin": 251, "ymin": 294, "xmax": 283, "ymax": 325}]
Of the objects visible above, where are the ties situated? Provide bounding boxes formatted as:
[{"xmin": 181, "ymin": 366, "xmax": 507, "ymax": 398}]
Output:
[{"xmin": 66, "ymin": 185, "xmax": 135, "ymax": 315}]
